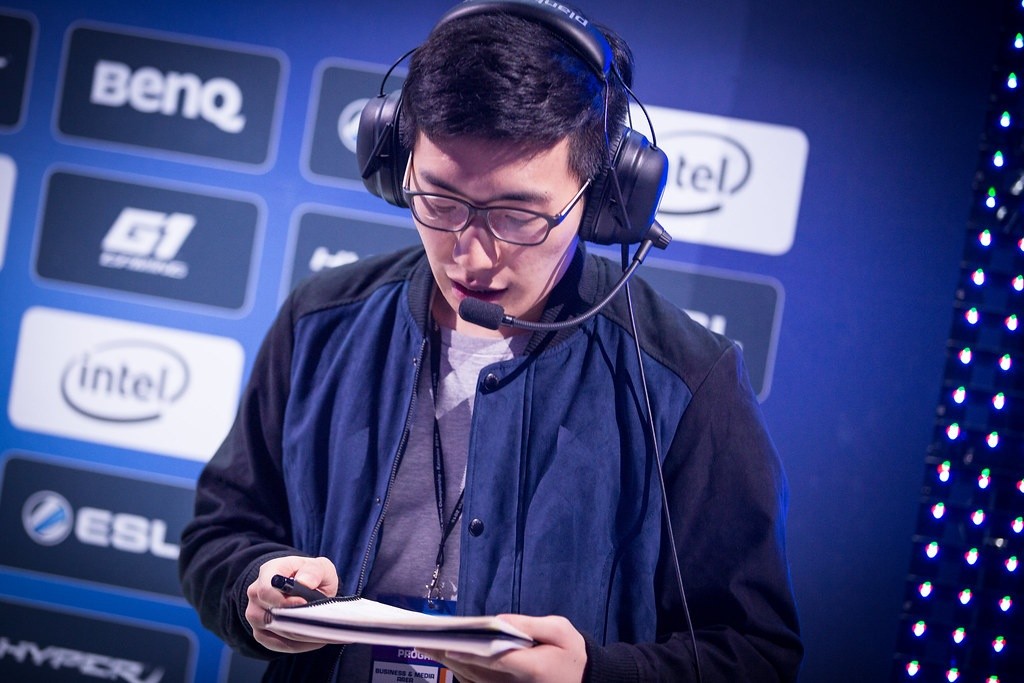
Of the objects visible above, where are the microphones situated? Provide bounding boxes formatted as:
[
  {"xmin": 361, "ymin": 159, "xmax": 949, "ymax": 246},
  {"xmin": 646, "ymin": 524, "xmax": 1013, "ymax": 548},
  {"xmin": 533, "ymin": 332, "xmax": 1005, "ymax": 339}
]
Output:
[{"xmin": 459, "ymin": 240, "xmax": 651, "ymax": 332}]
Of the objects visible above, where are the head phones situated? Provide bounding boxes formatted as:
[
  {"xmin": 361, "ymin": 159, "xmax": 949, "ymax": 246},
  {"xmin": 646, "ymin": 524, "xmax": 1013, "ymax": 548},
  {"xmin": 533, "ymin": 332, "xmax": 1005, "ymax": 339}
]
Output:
[{"xmin": 356, "ymin": 0, "xmax": 671, "ymax": 251}]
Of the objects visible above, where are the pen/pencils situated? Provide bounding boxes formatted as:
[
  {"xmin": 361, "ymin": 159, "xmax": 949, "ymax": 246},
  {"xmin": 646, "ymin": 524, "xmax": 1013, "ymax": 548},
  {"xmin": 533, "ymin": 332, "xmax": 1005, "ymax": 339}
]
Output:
[{"xmin": 271, "ymin": 574, "xmax": 327, "ymax": 601}]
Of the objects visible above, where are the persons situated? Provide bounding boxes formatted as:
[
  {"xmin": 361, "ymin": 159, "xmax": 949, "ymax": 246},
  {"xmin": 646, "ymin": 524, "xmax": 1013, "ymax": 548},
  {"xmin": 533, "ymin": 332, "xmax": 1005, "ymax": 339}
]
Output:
[{"xmin": 175, "ymin": 0, "xmax": 802, "ymax": 683}]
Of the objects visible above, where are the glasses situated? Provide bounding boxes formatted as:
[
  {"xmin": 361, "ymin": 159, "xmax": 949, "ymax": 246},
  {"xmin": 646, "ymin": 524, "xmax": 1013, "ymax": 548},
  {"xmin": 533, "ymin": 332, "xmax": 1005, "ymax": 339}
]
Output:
[{"xmin": 402, "ymin": 149, "xmax": 592, "ymax": 247}]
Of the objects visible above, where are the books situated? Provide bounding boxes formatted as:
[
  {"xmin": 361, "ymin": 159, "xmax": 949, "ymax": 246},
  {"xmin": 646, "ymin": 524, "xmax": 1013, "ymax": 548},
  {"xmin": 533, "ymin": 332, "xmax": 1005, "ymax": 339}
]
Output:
[{"xmin": 259, "ymin": 594, "xmax": 532, "ymax": 657}]
